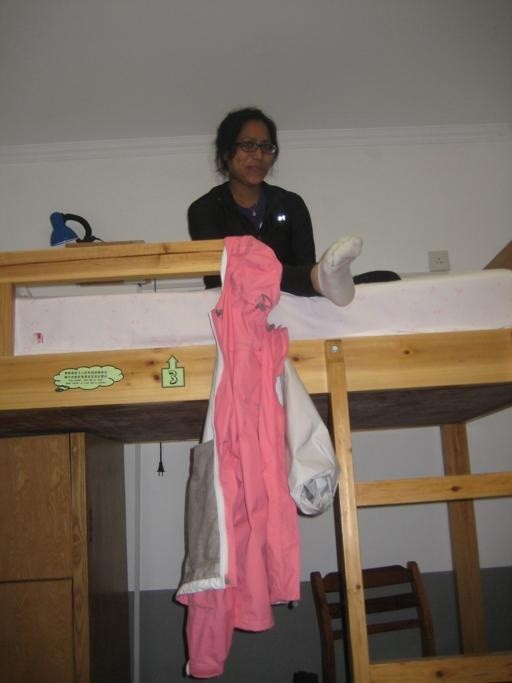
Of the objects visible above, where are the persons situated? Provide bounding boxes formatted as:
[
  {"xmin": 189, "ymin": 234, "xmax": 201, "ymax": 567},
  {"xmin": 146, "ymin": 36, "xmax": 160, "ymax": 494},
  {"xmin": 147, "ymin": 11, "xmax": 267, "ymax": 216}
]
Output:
[{"xmin": 188, "ymin": 108, "xmax": 401, "ymax": 307}]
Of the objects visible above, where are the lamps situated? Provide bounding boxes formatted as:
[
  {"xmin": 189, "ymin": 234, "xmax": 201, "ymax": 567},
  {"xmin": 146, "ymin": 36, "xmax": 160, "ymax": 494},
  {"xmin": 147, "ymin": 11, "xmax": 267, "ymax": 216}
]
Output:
[{"xmin": 47, "ymin": 212, "xmax": 96, "ymax": 245}]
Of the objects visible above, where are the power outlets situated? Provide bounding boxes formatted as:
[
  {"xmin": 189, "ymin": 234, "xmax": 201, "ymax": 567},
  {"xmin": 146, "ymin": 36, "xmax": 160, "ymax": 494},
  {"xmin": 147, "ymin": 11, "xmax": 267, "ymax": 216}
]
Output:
[{"xmin": 427, "ymin": 249, "xmax": 449, "ymax": 272}]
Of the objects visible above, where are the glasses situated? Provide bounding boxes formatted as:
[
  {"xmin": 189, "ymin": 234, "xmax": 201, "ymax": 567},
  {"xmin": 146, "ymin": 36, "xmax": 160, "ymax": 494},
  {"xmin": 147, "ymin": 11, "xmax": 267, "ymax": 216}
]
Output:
[{"xmin": 236, "ymin": 141, "xmax": 277, "ymax": 154}]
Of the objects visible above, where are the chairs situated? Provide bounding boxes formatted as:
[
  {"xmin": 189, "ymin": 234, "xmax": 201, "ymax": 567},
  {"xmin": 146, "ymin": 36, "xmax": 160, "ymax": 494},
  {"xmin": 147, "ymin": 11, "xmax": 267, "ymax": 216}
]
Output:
[{"xmin": 309, "ymin": 558, "xmax": 435, "ymax": 681}]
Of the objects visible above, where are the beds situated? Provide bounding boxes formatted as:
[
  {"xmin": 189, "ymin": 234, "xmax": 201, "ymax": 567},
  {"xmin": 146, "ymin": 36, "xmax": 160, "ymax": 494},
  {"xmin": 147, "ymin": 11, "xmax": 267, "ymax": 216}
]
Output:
[{"xmin": 1, "ymin": 235, "xmax": 512, "ymax": 683}]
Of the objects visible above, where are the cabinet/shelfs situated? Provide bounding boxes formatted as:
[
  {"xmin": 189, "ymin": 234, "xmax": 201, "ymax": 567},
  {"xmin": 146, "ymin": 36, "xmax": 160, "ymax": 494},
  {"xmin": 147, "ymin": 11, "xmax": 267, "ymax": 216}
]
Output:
[{"xmin": 0, "ymin": 432, "xmax": 132, "ymax": 683}]
